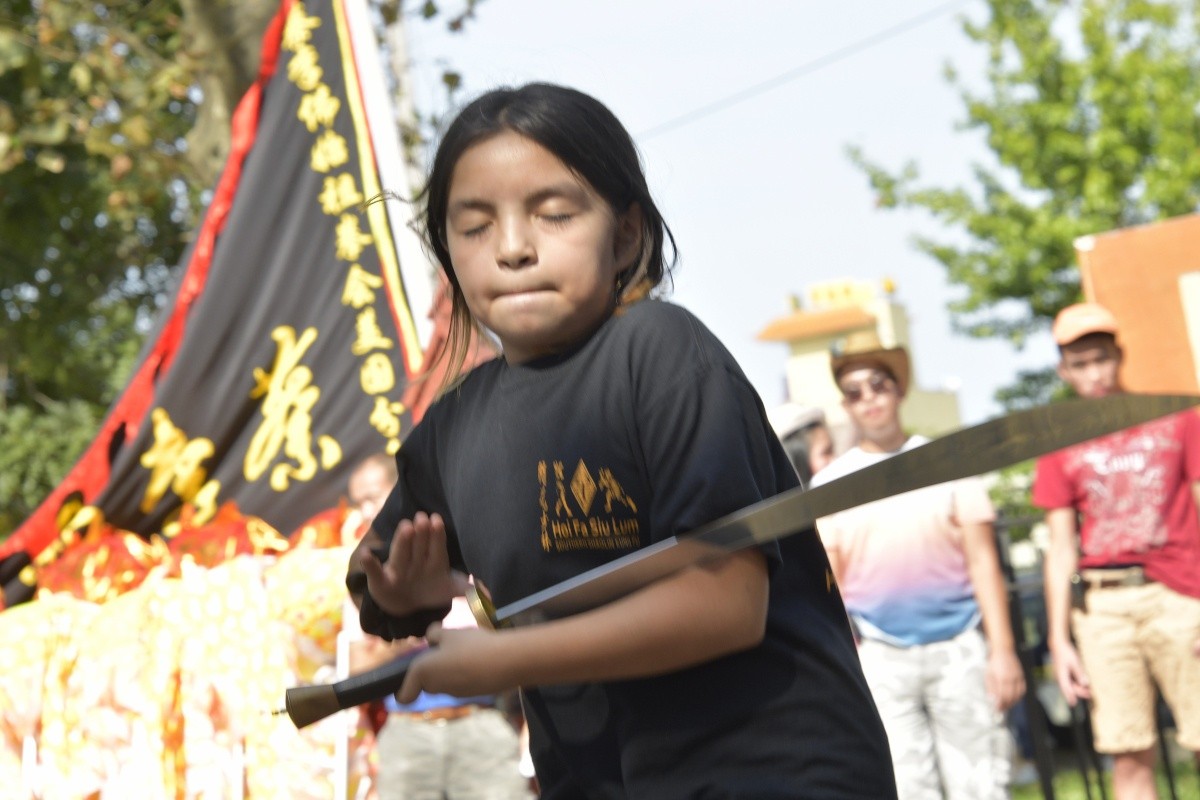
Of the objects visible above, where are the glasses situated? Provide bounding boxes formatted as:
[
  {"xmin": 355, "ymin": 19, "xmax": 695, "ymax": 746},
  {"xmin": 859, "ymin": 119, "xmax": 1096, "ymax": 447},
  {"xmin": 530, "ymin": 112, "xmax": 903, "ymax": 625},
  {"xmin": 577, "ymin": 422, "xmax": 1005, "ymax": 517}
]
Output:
[{"xmin": 847, "ymin": 372, "xmax": 888, "ymax": 401}]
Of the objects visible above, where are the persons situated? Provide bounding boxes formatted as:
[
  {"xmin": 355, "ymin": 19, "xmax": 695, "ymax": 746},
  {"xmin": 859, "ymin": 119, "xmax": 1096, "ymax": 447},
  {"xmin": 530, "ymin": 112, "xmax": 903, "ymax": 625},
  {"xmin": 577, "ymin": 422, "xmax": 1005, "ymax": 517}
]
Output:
[
  {"xmin": 769, "ymin": 329, "xmax": 1027, "ymax": 800},
  {"xmin": 346, "ymin": 83, "xmax": 899, "ymax": 800},
  {"xmin": 1043, "ymin": 304, "xmax": 1200, "ymax": 800},
  {"xmin": 769, "ymin": 403, "xmax": 844, "ymax": 486},
  {"xmin": 346, "ymin": 453, "xmax": 546, "ymax": 800}
]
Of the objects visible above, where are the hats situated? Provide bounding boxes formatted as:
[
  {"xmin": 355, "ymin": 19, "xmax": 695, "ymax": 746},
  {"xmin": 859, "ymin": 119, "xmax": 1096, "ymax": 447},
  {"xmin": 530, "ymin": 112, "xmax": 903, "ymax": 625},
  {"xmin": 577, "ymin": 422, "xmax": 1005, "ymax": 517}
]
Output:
[
  {"xmin": 769, "ymin": 405, "xmax": 826, "ymax": 441},
  {"xmin": 1052, "ymin": 304, "xmax": 1117, "ymax": 346},
  {"xmin": 829, "ymin": 330, "xmax": 908, "ymax": 398}
]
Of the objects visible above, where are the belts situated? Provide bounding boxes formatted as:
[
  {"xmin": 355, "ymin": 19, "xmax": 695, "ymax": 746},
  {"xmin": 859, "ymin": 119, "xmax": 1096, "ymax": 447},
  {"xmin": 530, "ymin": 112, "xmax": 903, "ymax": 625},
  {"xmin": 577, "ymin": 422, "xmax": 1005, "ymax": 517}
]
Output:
[
  {"xmin": 1081, "ymin": 576, "xmax": 1154, "ymax": 590},
  {"xmin": 385, "ymin": 704, "xmax": 493, "ymax": 719}
]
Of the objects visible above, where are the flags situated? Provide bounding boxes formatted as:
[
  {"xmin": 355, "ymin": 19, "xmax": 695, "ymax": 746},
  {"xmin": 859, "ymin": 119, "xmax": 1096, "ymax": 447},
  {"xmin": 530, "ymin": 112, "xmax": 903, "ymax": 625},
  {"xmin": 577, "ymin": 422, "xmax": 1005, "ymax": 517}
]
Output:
[{"xmin": 1, "ymin": 1, "xmax": 427, "ymax": 616}]
